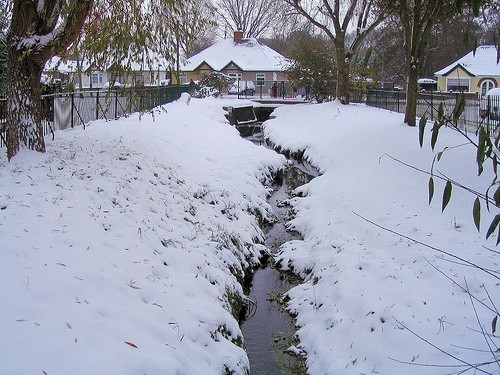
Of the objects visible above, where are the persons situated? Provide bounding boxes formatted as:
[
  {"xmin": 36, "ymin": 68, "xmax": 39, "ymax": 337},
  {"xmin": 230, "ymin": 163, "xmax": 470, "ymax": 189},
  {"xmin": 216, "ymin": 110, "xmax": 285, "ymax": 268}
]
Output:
[{"xmin": 188, "ymin": 78, "xmax": 196, "ymax": 86}]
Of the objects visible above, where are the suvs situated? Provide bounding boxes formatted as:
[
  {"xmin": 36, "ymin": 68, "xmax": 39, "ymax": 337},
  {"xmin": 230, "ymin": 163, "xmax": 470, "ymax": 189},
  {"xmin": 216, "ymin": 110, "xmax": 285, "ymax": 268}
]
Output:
[
  {"xmin": 268, "ymin": 82, "xmax": 295, "ymax": 98},
  {"xmin": 229, "ymin": 81, "xmax": 257, "ymax": 95}
]
